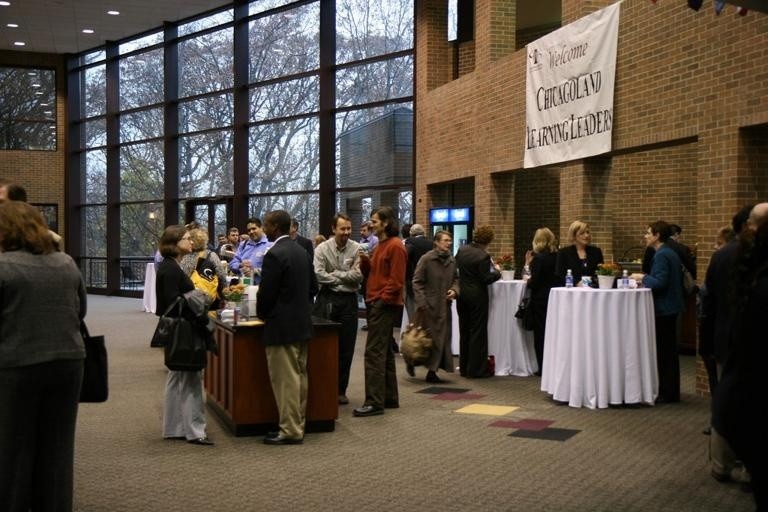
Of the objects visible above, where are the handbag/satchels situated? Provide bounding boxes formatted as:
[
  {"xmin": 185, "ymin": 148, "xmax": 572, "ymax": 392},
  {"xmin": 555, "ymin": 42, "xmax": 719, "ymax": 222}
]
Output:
[
  {"xmin": 189, "ymin": 251, "xmax": 218, "ymax": 304},
  {"xmin": 151, "ymin": 296, "xmax": 181, "ymax": 348},
  {"xmin": 515, "ymin": 280, "xmax": 534, "ymax": 331},
  {"xmin": 400, "ymin": 309, "xmax": 433, "ymax": 365},
  {"xmin": 79, "ymin": 320, "xmax": 109, "ymax": 403},
  {"xmin": 679, "ymin": 260, "xmax": 697, "ymax": 296}
]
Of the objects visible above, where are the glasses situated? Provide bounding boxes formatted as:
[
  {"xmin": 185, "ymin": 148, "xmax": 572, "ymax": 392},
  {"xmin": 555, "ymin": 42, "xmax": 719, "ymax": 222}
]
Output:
[{"xmin": 441, "ymin": 238, "xmax": 453, "ymax": 243}]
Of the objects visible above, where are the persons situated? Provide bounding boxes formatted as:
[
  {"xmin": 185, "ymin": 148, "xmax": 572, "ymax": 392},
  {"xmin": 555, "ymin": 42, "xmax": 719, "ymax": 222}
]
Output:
[
  {"xmin": 352, "ymin": 206, "xmax": 406, "ymax": 417},
  {"xmin": 180, "ymin": 228, "xmax": 223, "ymax": 310},
  {"xmin": 220, "ymin": 227, "xmax": 240, "ymax": 262},
  {"xmin": 358, "ymin": 222, "xmax": 379, "ymax": 331},
  {"xmin": 404, "ymin": 224, "xmax": 433, "ymax": 323},
  {"xmin": 313, "ymin": 236, "xmax": 326, "ymax": 249},
  {"xmin": 401, "ymin": 224, "xmax": 412, "ymax": 245},
  {"xmin": 1, "ymin": 183, "xmax": 61, "ymax": 251},
  {"xmin": 155, "ymin": 226, "xmax": 217, "ymax": 445},
  {"xmin": 627, "ymin": 220, "xmax": 688, "ymax": 403},
  {"xmin": 0, "ymin": 200, "xmax": 87, "ymax": 511},
  {"xmin": 698, "ymin": 202, "xmax": 768, "ymax": 512},
  {"xmin": 523, "ymin": 228, "xmax": 568, "ymax": 377},
  {"xmin": 455, "ymin": 225, "xmax": 501, "ymax": 378},
  {"xmin": 642, "ymin": 224, "xmax": 698, "ymax": 281},
  {"xmin": 314, "ymin": 213, "xmax": 363, "ymax": 404},
  {"xmin": 230, "ymin": 217, "xmax": 272, "ymax": 284},
  {"xmin": 559, "ymin": 220, "xmax": 605, "ymax": 287},
  {"xmin": 240, "ymin": 233, "xmax": 251, "ymax": 244},
  {"xmin": 288, "ymin": 218, "xmax": 318, "ymax": 304},
  {"xmin": 404, "ymin": 231, "xmax": 460, "ymax": 384},
  {"xmin": 256, "ymin": 210, "xmax": 312, "ymax": 445},
  {"xmin": 217, "ymin": 234, "xmax": 228, "ymax": 261}
]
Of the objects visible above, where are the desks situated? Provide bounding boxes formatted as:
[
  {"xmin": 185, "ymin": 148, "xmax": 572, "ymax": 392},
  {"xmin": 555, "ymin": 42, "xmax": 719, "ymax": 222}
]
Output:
[
  {"xmin": 487, "ymin": 278, "xmax": 539, "ymax": 376},
  {"xmin": 207, "ymin": 310, "xmax": 342, "ymax": 436},
  {"xmin": 540, "ymin": 287, "xmax": 659, "ymax": 409}
]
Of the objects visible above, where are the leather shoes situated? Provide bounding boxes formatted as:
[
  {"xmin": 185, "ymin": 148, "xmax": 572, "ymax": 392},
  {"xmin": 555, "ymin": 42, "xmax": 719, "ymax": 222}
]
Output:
[
  {"xmin": 266, "ymin": 431, "xmax": 279, "ymax": 438},
  {"xmin": 263, "ymin": 436, "xmax": 304, "ymax": 445},
  {"xmin": 426, "ymin": 371, "xmax": 445, "ymax": 383},
  {"xmin": 408, "ymin": 366, "xmax": 415, "ymax": 376},
  {"xmin": 339, "ymin": 395, "xmax": 349, "ymax": 404},
  {"xmin": 189, "ymin": 438, "xmax": 215, "ymax": 445},
  {"xmin": 385, "ymin": 404, "xmax": 400, "ymax": 408},
  {"xmin": 352, "ymin": 406, "xmax": 384, "ymax": 417},
  {"xmin": 164, "ymin": 437, "xmax": 186, "ymax": 441}
]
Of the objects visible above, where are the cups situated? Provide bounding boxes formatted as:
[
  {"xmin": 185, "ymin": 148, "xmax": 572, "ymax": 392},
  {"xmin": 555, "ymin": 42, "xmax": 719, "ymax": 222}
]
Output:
[
  {"xmin": 581, "ymin": 276, "xmax": 592, "ymax": 289},
  {"xmin": 360, "ymin": 243, "xmax": 369, "ymax": 256}
]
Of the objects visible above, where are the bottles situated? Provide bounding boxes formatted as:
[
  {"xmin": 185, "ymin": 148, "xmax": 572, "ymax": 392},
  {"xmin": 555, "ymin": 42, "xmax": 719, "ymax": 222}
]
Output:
[
  {"xmin": 565, "ymin": 269, "xmax": 574, "ymax": 288},
  {"xmin": 622, "ymin": 269, "xmax": 629, "ymax": 290},
  {"xmin": 241, "ymin": 294, "xmax": 250, "ymax": 321}
]
